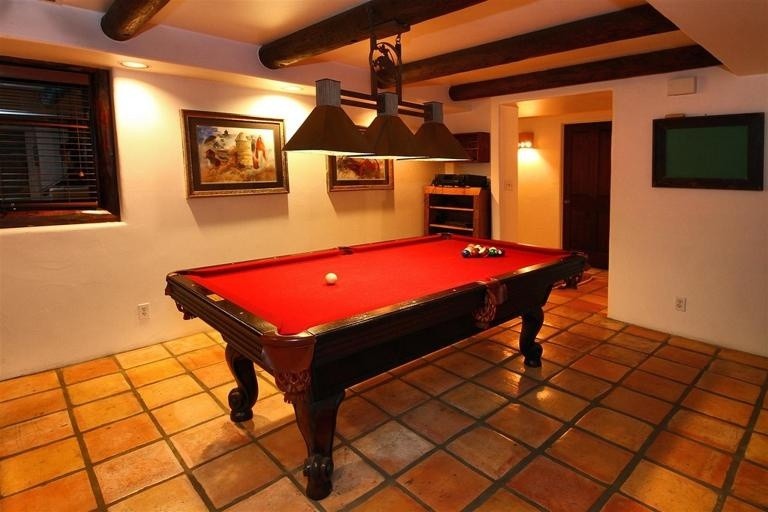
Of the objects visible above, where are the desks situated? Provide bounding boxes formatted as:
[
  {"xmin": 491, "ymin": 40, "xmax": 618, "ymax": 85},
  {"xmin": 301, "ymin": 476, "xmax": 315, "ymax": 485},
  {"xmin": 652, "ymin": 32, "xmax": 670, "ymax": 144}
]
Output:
[{"xmin": 164, "ymin": 235, "xmax": 576, "ymax": 502}]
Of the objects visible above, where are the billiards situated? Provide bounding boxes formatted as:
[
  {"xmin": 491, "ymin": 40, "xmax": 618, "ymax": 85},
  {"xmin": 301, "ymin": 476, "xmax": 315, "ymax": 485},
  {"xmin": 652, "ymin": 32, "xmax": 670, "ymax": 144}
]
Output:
[
  {"xmin": 463, "ymin": 242, "xmax": 504, "ymax": 257},
  {"xmin": 325, "ymin": 273, "xmax": 338, "ymax": 285}
]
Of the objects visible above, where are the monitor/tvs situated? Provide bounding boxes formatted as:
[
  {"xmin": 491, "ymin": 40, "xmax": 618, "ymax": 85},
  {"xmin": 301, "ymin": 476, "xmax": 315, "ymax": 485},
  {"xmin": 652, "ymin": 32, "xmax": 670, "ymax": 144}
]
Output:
[{"xmin": 653, "ymin": 112, "xmax": 765, "ymax": 191}]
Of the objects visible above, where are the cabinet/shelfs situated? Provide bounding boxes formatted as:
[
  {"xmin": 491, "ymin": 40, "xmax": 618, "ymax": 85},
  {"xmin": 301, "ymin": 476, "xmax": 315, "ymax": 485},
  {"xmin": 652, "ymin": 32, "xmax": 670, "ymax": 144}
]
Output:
[{"xmin": 421, "ymin": 184, "xmax": 488, "ymax": 243}]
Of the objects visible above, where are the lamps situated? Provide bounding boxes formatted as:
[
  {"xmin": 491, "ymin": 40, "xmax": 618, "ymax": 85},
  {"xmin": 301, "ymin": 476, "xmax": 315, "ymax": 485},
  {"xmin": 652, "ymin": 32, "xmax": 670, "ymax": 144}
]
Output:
[{"xmin": 279, "ymin": 27, "xmax": 475, "ymax": 166}]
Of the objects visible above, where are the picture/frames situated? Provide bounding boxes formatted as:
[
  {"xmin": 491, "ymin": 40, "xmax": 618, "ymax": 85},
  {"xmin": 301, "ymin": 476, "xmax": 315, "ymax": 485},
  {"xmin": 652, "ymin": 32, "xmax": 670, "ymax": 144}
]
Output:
[
  {"xmin": 323, "ymin": 124, "xmax": 395, "ymax": 192},
  {"xmin": 179, "ymin": 108, "xmax": 290, "ymax": 198}
]
